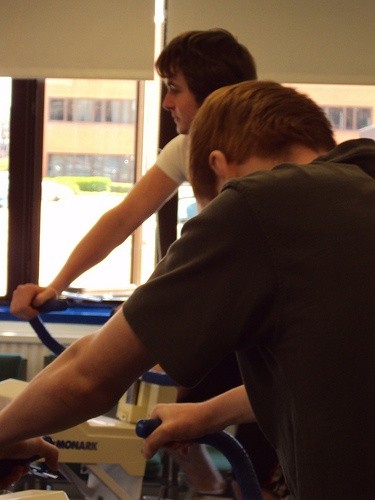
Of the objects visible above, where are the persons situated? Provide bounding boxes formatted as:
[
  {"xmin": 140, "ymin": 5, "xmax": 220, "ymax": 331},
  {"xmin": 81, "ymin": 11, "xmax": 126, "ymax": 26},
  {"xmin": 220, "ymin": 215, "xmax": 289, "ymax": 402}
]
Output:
[
  {"xmin": 1, "ymin": 79, "xmax": 375, "ymax": 499},
  {"xmin": 9, "ymin": 28, "xmax": 290, "ymax": 500}
]
